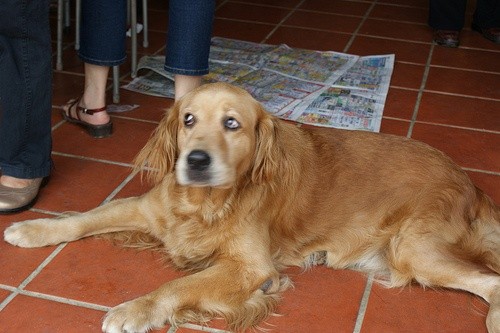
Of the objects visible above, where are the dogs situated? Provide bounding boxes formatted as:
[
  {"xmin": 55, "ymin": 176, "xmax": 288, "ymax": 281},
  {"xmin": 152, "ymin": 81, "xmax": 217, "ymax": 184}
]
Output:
[{"xmin": 2, "ymin": 81, "xmax": 500, "ymax": 333}]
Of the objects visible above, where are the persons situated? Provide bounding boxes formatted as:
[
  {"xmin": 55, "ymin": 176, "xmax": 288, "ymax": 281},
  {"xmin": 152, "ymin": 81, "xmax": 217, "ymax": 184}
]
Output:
[
  {"xmin": 427, "ymin": 0, "xmax": 500, "ymax": 48},
  {"xmin": 62, "ymin": 0, "xmax": 216, "ymax": 139},
  {"xmin": 0, "ymin": 0, "xmax": 55, "ymax": 214}
]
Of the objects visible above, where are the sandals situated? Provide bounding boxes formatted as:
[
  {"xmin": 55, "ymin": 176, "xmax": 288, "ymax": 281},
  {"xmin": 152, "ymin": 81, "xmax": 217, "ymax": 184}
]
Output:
[{"xmin": 63, "ymin": 99, "xmax": 112, "ymax": 140}]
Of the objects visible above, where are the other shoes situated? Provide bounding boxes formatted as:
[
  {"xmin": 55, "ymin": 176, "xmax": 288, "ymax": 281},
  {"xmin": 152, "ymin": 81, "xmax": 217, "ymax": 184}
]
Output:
[
  {"xmin": 0, "ymin": 174, "xmax": 50, "ymax": 213},
  {"xmin": 428, "ymin": 26, "xmax": 459, "ymax": 48},
  {"xmin": 472, "ymin": 24, "xmax": 500, "ymax": 42}
]
